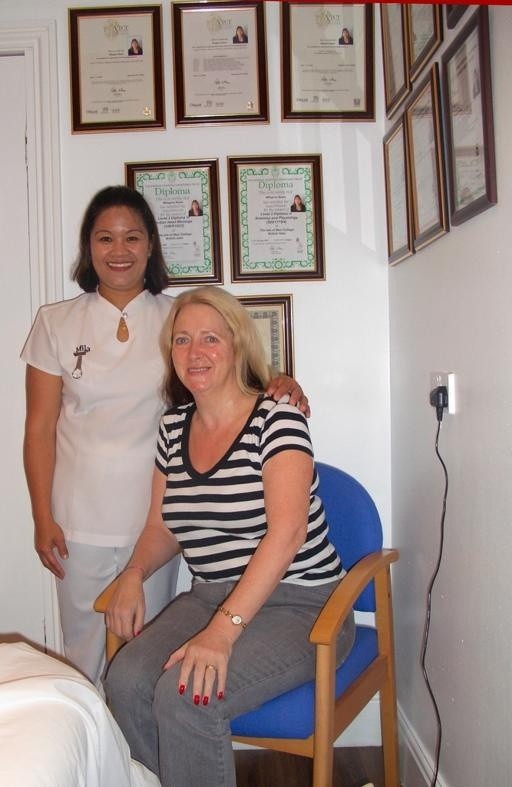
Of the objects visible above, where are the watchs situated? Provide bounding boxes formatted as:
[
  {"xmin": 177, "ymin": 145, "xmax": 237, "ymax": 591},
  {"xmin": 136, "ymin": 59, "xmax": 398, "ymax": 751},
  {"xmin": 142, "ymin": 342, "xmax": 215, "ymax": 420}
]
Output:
[{"xmin": 218, "ymin": 606, "xmax": 247, "ymax": 629}]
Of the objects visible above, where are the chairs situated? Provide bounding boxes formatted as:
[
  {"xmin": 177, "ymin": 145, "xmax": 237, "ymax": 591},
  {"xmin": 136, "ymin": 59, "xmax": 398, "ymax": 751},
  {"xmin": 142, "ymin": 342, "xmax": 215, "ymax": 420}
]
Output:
[{"xmin": 94, "ymin": 461, "xmax": 401, "ymax": 787}]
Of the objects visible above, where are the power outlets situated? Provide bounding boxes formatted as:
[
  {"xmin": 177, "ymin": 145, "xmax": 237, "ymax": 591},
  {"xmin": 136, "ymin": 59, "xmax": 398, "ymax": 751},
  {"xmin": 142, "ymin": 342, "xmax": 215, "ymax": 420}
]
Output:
[{"xmin": 435, "ymin": 373, "xmax": 456, "ymax": 414}]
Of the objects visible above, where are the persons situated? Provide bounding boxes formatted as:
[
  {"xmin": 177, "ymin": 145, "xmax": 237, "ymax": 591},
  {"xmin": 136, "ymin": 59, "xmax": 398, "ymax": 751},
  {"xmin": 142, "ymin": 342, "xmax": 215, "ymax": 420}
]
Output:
[
  {"xmin": 291, "ymin": 195, "xmax": 306, "ymax": 212},
  {"xmin": 339, "ymin": 29, "xmax": 353, "ymax": 45},
  {"xmin": 128, "ymin": 39, "xmax": 143, "ymax": 55},
  {"xmin": 100, "ymin": 286, "xmax": 356, "ymax": 787},
  {"xmin": 473, "ymin": 70, "xmax": 482, "ymax": 98},
  {"xmin": 233, "ymin": 26, "xmax": 248, "ymax": 43},
  {"xmin": 189, "ymin": 200, "xmax": 203, "ymax": 215},
  {"xmin": 20, "ymin": 187, "xmax": 311, "ymax": 703}
]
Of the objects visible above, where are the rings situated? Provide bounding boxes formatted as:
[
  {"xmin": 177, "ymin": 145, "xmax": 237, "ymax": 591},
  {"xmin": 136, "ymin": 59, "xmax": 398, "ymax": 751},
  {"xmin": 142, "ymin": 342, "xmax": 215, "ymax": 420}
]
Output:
[{"xmin": 206, "ymin": 665, "xmax": 216, "ymax": 670}]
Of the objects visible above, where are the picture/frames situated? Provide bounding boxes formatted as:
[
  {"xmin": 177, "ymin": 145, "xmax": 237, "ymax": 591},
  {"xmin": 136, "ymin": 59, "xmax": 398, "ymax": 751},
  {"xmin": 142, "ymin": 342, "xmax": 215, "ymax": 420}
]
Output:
[
  {"xmin": 280, "ymin": 1, "xmax": 376, "ymax": 123},
  {"xmin": 227, "ymin": 155, "xmax": 328, "ymax": 282},
  {"xmin": 67, "ymin": 4, "xmax": 166, "ymax": 135},
  {"xmin": 124, "ymin": 158, "xmax": 224, "ymax": 287},
  {"xmin": 171, "ymin": 0, "xmax": 270, "ymax": 127},
  {"xmin": 219, "ymin": 294, "xmax": 295, "ymax": 380},
  {"xmin": 379, "ymin": 5, "xmax": 496, "ymax": 265}
]
